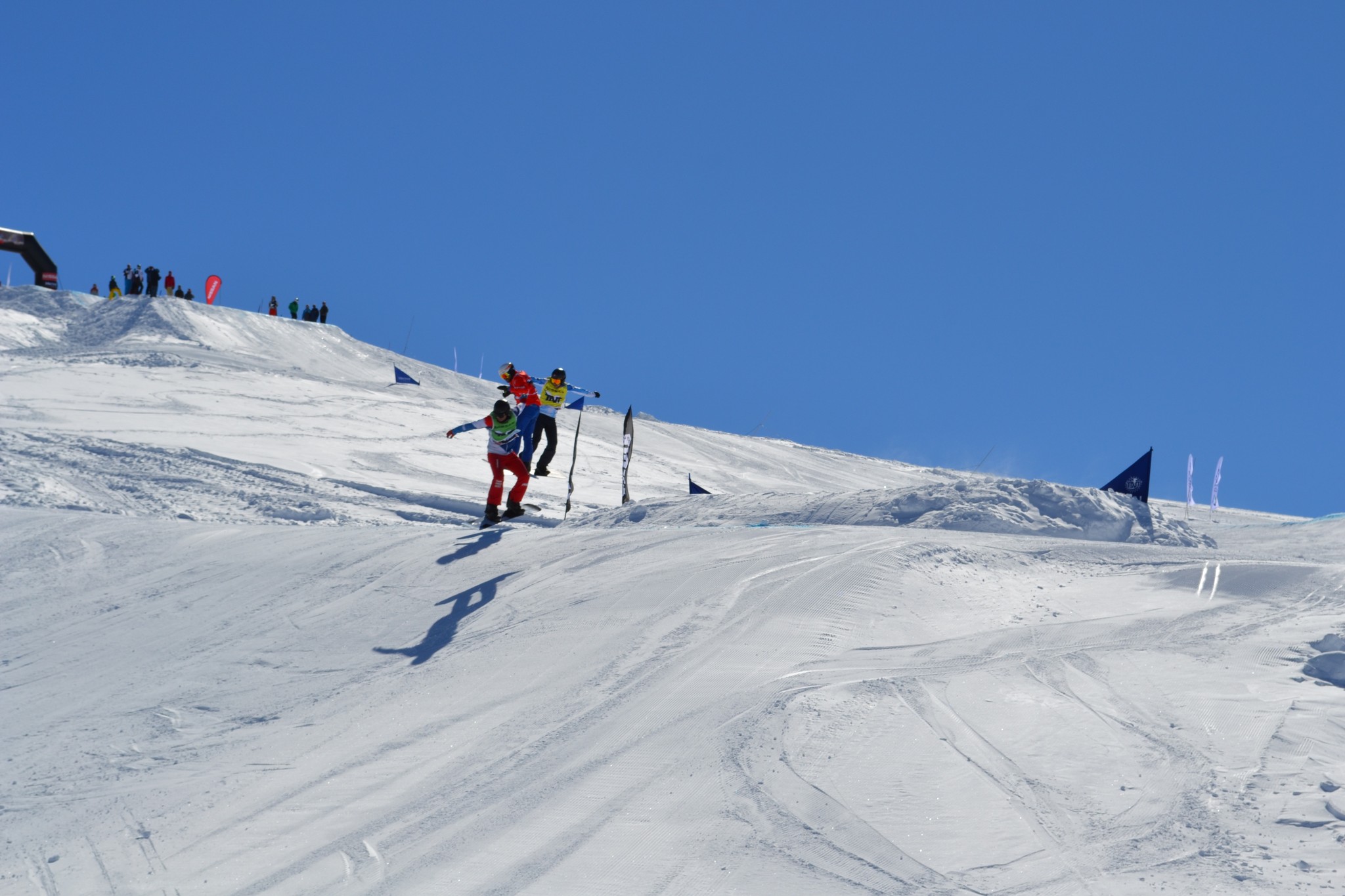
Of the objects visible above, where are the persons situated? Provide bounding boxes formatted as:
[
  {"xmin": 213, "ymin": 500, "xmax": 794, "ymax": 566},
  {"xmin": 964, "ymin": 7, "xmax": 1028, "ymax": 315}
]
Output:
[
  {"xmin": 269, "ymin": 296, "xmax": 278, "ymax": 316},
  {"xmin": 519, "ymin": 368, "xmax": 601, "ymax": 475},
  {"xmin": 90, "ymin": 284, "xmax": 99, "ymax": 295},
  {"xmin": 319, "ymin": 301, "xmax": 329, "ymax": 324},
  {"xmin": 145, "ymin": 266, "xmax": 161, "ymax": 297},
  {"xmin": 288, "ymin": 297, "xmax": 299, "ymax": 319},
  {"xmin": 123, "ymin": 264, "xmax": 144, "ymax": 295},
  {"xmin": 164, "ymin": 271, "xmax": 175, "ymax": 297},
  {"xmin": 109, "ymin": 275, "xmax": 122, "ymax": 300},
  {"xmin": 184, "ymin": 288, "xmax": 195, "ymax": 300},
  {"xmin": 175, "ymin": 285, "xmax": 184, "ymax": 298},
  {"xmin": 302, "ymin": 304, "xmax": 319, "ymax": 322},
  {"xmin": 497, "ymin": 361, "xmax": 542, "ymax": 471},
  {"xmin": 446, "ymin": 394, "xmax": 530, "ymax": 522}
]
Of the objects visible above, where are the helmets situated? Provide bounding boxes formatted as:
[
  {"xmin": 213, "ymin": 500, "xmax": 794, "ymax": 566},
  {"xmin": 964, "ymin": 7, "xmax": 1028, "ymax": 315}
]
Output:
[
  {"xmin": 498, "ymin": 363, "xmax": 512, "ymax": 375},
  {"xmin": 494, "ymin": 400, "xmax": 510, "ymax": 414},
  {"xmin": 552, "ymin": 368, "xmax": 566, "ymax": 380}
]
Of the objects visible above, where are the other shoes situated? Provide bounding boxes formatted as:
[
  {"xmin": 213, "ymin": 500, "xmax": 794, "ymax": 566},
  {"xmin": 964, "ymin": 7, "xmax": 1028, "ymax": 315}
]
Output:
[
  {"xmin": 506, "ymin": 503, "xmax": 520, "ymax": 516},
  {"xmin": 535, "ymin": 466, "xmax": 550, "ymax": 476},
  {"xmin": 485, "ymin": 504, "xmax": 497, "ymax": 518}
]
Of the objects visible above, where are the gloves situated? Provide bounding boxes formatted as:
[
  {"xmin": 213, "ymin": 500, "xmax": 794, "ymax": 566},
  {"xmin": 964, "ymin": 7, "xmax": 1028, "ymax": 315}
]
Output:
[{"xmin": 594, "ymin": 391, "xmax": 601, "ymax": 398}]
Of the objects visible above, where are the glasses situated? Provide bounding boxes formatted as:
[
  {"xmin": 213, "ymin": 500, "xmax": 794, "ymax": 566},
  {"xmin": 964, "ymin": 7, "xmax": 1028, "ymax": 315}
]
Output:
[
  {"xmin": 551, "ymin": 378, "xmax": 561, "ymax": 385},
  {"xmin": 501, "ymin": 372, "xmax": 510, "ymax": 380},
  {"xmin": 496, "ymin": 413, "xmax": 504, "ymax": 419}
]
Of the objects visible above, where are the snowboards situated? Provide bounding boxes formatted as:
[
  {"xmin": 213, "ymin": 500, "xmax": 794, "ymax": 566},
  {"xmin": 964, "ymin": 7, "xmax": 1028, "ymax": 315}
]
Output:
[
  {"xmin": 481, "ymin": 458, "xmax": 538, "ymax": 479},
  {"xmin": 461, "ymin": 504, "xmax": 541, "ymax": 530},
  {"xmin": 530, "ymin": 469, "xmax": 566, "ymax": 479}
]
